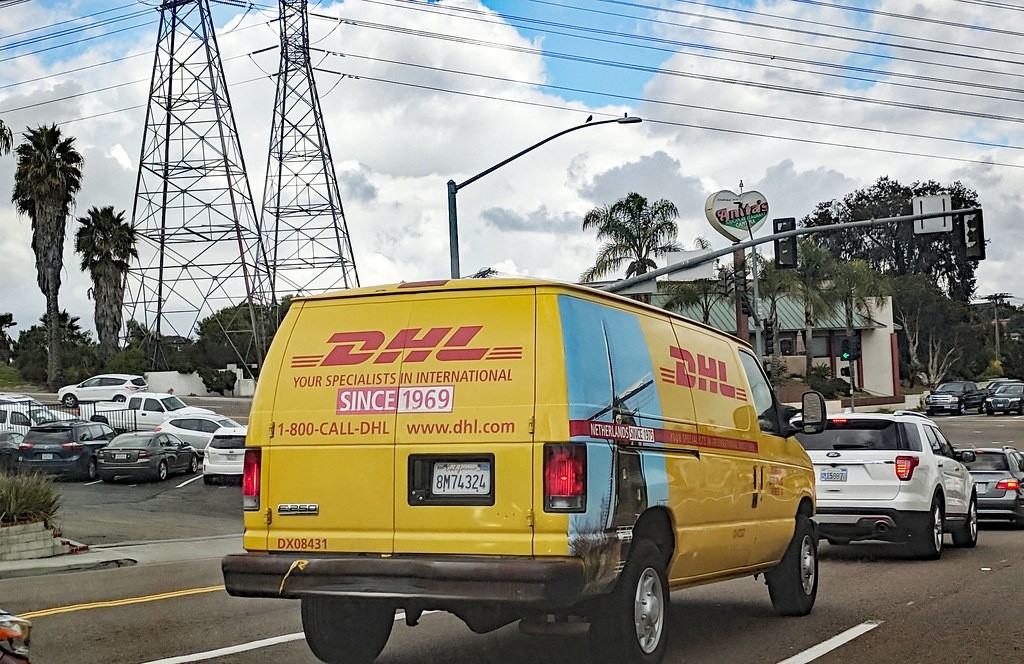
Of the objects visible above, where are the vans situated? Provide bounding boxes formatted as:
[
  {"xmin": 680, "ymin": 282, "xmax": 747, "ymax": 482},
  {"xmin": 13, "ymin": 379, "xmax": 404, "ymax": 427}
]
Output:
[
  {"xmin": 222, "ymin": 277, "xmax": 826, "ymax": 664},
  {"xmin": 0, "ymin": 403, "xmax": 61, "ymax": 438}
]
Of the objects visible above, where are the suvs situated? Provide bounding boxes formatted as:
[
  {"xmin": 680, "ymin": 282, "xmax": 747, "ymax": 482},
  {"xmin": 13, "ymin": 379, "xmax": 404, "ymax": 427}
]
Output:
[
  {"xmin": 17, "ymin": 417, "xmax": 120, "ymax": 482},
  {"xmin": 202, "ymin": 426, "xmax": 249, "ymax": 487},
  {"xmin": 789, "ymin": 410, "xmax": 982, "ymax": 562},
  {"xmin": 58, "ymin": 373, "xmax": 150, "ymax": 409},
  {"xmin": 952, "ymin": 443, "xmax": 1024, "ymax": 531}
]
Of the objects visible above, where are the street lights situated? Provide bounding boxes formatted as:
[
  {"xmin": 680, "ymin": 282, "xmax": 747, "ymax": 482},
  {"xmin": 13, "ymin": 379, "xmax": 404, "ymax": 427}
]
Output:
[{"xmin": 445, "ymin": 110, "xmax": 642, "ymax": 282}]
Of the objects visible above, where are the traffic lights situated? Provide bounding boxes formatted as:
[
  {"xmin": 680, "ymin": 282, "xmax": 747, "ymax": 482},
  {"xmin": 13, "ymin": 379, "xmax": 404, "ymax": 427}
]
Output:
[
  {"xmin": 851, "ymin": 334, "xmax": 861, "ymax": 361},
  {"xmin": 737, "ymin": 268, "xmax": 748, "ymax": 295},
  {"xmin": 764, "ymin": 317, "xmax": 774, "ymax": 356},
  {"xmin": 839, "ymin": 336, "xmax": 851, "ymax": 361},
  {"xmin": 741, "ymin": 292, "xmax": 752, "ymax": 317},
  {"xmin": 717, "ymin": 270, "xmax": 728, "ymax": 296},
  {"xmin": 841, "ymin": 367, "xmax": 851, "ymax": 375}
]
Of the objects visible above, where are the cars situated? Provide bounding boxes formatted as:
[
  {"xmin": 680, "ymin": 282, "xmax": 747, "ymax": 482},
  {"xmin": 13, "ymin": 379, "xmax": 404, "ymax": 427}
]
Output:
[
  {"xmin": 155, "ymin": 413, "xmax": 244, "ymax": 459},
  {"xmin": 977, "ymin": 378, "xmax": 1023, "ymax": 396},
  {"xmin": 986, "ymin": 382, "xmax": 1024, "ymax": 417},
  {"xmin": 0, "ymin": 431, "xmax": 26, "ymax": 472},
  {"xmin": 0, "ymin": 392, "xmax": 78, "ymax": 421},
  {"xmin": 96, "ymin": 431, "xmax": 201, "ymax": 483}
]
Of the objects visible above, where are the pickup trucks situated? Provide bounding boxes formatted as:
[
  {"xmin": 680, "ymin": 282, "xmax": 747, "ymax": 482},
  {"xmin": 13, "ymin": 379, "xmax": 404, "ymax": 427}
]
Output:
[
  {"xmin": 924, "ymin": 380, "xmax": 989, "ymax": 415},
  {"xmin": 78, "ymin": 391, "xmax": 216, "ymax": 433}
]
